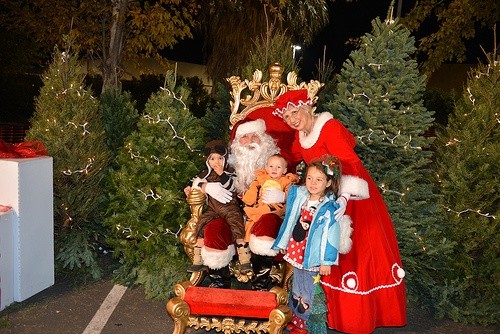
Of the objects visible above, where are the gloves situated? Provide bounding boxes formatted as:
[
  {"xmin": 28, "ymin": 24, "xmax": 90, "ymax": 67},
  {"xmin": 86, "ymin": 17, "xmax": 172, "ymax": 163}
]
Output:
[
  {"xmin": 262, "ymin": 188, "xmax": 285, "ymax": 204},
  {"xmin": 334, "ymin": 193, "xmax": 348, "ymax": 223},
  {"xmin": 204, "ymin": 182, "xmax": 233, "ymax": 204}
]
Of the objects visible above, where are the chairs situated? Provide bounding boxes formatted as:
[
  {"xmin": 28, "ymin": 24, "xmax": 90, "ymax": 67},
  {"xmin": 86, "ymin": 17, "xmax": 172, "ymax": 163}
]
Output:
[{"xmin": 165, "ymin": 62, "xmax": 325, "ymax": 334}]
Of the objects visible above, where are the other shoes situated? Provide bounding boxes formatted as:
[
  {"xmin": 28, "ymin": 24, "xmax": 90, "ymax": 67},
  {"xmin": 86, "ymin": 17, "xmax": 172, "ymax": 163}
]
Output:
[
  {"xmin": 286, "ymin": 315, "xmax": 306, "ymax": 332},
  {"xmin": 240, "ymin": 262, "xmax": 252, "ymax": 272},
  {"xmin": 186, "ymin": 263, "xmax": 207, "ymax": 273},
  {"xmin": 236, "ymin": 232, "xmax": 279, "ymax": 291},
  {"xmin": 199, "ymin": 244, "xmax": 232, "ymax": 290}
]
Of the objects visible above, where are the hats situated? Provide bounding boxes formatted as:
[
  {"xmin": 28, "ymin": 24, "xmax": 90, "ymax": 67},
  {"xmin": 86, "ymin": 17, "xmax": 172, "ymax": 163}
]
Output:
[
  {"xmin": 246, "ymin": 213, "xmax": 285, "ymax": 257},
  {"xmin": 200, "ymin": 218, "xmax": 235, "ymax": 271},
  {"xmin": 203, "ymin": 138, "xmax": 229, "ymax": 178},
  {"xmin": 272, "ymin": 89, "xmax": 314, "ymax": 118},
  {"xmin": 228, "ymin": 115, "xmax": 267, "ymax": 148}
]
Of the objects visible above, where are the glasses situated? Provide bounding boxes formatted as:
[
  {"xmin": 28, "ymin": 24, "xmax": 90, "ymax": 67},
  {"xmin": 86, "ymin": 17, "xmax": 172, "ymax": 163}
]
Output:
[
  {"xmin": 242, "ymin": 134, "xmax": 258, "ymax": 139},
  {"xmin": 284, "ymin": 108, "xmax": 301, "ymax": 123}
]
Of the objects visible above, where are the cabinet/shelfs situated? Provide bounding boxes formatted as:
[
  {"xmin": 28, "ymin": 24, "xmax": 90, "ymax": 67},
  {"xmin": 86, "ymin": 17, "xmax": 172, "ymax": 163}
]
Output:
[{"xmin": 0, "ymin": 154, "xmax": 55, "ymax": 314}]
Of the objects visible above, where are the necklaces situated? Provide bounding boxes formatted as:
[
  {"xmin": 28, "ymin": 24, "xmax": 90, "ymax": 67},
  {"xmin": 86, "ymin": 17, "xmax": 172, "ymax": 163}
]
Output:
[{"xmin": 311, "ymin": 116, "xmax": 312, "ymax": 132}]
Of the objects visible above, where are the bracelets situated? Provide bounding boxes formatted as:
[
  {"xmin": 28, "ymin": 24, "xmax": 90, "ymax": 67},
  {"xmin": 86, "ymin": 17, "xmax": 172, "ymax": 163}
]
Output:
[{"xmin": 342, "ymin": 196, "xmax": 347, "ymax": 201}]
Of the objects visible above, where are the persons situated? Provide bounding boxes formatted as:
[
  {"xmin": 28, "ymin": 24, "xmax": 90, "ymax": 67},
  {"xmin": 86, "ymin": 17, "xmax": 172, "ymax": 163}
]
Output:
[
  {"xmin": 273, "ymin": 90, "xmax": 407, "ymax": 334},
  {"xmin": 242, "ymin": 155, "xmax": 292, "ymax": 249},
  {"xmin": 183, "ymin": 118, "xmax": 287, "ymax": 290},
  {"xmin": 187, "ymin": 141, "xmax": 252, "ymax": 274},
  {"xmin": 271, "ymin": 155, "xmax": 341, "ymax": 314}
]
